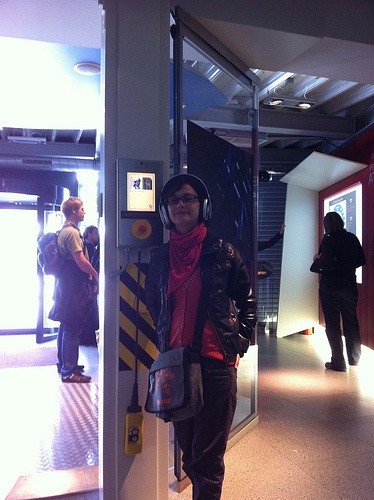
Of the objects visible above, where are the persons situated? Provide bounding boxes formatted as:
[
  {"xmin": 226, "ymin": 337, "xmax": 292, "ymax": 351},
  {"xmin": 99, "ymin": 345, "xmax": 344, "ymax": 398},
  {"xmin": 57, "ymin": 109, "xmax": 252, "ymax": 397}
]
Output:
[
  {"xmin": 79, "ymin": 226, "xmax": 100, "ymax": 347},
  {"xmin": 313, "ymin": 212, "xmax": 367, "ymax": 372},
  {"xmin": 258, "ymin": 223, "xmax": 286, "ymax": 252},
  {"xmin": 55, "ymin": 196, "xmax": 98, "ymax": 383},
  {"xmin": 145, "ymin": 173, "xmax": 258, "ymax": 500}
]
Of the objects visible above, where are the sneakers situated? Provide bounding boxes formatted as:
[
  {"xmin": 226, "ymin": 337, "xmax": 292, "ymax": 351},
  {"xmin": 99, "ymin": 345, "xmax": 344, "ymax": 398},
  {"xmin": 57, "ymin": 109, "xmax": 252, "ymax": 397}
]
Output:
[
  {"xmin": 58, "ymin": 366, "xmax": 84, "ymax": 373},
  {"xmin": 62, "ymin": 373, "xmax": 91, "ymax": 383}
]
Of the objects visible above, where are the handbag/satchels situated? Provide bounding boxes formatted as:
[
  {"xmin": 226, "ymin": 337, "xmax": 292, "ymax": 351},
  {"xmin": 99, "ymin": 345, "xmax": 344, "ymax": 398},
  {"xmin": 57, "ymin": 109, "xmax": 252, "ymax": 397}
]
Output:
[
  {"xmin": 310, "ymin": 258, "xmax": 334, "ymax": 275},
  {"xmin": 145, "ymin": 348, "xmax": 204, "ymax": 422}
]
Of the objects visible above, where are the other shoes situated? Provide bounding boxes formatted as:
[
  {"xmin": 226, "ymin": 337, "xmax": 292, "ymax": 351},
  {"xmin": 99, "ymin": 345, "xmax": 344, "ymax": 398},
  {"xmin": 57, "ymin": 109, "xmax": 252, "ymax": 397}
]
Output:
[
  {"xmin": 349, "ymin": 362, "xmax": 356, "ymax": 365},
  {"xmin": 325, "ymin": 362, "xmax": 346, "ymax": 372}
]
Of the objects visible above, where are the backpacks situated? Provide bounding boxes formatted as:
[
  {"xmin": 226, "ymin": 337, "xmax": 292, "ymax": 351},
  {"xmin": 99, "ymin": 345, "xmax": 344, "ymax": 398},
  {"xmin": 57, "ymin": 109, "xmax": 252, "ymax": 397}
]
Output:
[{"xmin": 38, "ymin": 224, "xmax": 82, "ymax": 275}]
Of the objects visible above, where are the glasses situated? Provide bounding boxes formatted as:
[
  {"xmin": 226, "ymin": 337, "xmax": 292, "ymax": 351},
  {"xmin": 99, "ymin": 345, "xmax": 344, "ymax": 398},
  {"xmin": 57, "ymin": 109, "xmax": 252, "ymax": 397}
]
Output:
[{"xmin": 165, "ymin": 194, "xmax": 201, "ymax": 204}]
